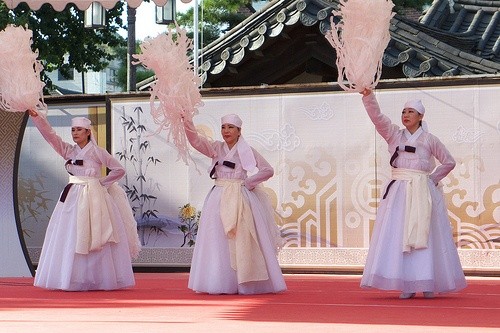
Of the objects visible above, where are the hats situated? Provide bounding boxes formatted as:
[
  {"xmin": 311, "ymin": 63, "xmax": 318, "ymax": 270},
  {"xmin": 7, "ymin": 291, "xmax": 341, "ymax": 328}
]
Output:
[
  {"xmin": 71, "ymin": 117, "xmax": 92, "ymax": 129},
  {"xmin": 221, "ymin": 114, "xmax": 242, "ymax": 128},
  {"xmin": 403, "ymin": 99, "xmax": 425, "ymax": 115}
]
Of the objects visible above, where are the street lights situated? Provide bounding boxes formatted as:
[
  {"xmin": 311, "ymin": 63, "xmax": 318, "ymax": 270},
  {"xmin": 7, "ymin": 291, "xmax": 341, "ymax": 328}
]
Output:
[{"xmin": 83, "ymin": 0, "xmax": 177, "ymax": 92}]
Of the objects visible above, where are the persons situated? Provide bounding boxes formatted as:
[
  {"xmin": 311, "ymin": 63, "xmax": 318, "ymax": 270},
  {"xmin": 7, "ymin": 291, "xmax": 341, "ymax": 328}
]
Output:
[
  {"xmin": 28, "ymin": 108, "xmax": 139, "ymax": 292},
  {"xmin": 179, "ymin": 108, "xmax": 287, "ymax": 295},
  {"xmin": 359, "ymin": 87, "xmax": 466, "ymax": 300}
]
